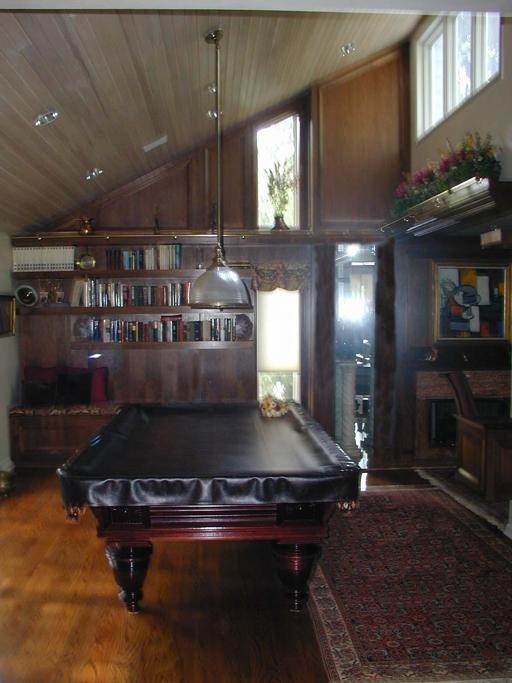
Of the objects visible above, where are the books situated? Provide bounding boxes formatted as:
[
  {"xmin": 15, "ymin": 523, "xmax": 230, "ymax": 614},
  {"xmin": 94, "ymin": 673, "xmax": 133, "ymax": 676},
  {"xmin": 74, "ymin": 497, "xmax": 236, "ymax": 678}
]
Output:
[{"xmin": 72, "ymin": 245, "xmax": 237, "ymax": 342}]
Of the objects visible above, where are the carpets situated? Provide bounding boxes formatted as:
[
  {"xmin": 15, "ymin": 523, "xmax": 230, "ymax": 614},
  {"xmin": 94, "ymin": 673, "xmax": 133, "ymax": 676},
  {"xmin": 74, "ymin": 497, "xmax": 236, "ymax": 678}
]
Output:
[{"xmin": 298, "ymin": 482, "xmax": 512, "ymax": 683}]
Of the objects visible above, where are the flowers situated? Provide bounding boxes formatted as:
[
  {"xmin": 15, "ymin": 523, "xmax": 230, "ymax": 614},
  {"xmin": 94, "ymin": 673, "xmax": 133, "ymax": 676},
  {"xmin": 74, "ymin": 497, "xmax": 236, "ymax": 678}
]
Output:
[{"xmin": 393, "ymin": 128, "xmax": 505, "ymax": 200}]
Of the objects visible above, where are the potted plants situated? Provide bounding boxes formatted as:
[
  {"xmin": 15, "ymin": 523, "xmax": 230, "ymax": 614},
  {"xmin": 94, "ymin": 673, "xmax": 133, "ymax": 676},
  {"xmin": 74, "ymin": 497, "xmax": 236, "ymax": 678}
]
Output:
[{"xmin": 262, "ymin": 157, "xmax": 299, "ymax": 232}]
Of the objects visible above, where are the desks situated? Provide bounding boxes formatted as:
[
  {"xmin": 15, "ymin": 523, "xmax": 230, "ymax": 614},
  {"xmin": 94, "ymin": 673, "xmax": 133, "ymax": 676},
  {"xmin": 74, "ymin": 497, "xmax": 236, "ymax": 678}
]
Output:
[{"xmin": 56, "ymin": 393, "xmax": 367, "ymax": 615}]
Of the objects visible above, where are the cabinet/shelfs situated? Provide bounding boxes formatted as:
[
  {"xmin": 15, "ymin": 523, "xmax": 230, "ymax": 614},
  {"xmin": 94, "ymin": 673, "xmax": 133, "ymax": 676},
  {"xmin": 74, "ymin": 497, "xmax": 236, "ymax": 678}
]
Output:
[
  {"xmin": 12, "ymin": 243, "xmax": 258, "ymax": 352},
  {"xmin": 8, "ymin": 403, "xmax": 121, "ymax": 470}
]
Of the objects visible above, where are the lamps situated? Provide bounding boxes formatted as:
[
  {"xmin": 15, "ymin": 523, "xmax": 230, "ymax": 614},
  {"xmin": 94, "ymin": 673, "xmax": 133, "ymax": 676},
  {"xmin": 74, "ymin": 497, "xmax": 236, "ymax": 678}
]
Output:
[{"xmin": 188, "ymin": 25, "xmax": 259, "ymax": 313}]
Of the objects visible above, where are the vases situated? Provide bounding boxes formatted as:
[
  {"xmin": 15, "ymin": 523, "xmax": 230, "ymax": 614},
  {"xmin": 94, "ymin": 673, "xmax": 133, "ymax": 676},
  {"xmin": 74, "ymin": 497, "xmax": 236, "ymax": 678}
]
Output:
[{"xmin": 388, "ymin": 175, "xmax": 504, "ymax": 237}]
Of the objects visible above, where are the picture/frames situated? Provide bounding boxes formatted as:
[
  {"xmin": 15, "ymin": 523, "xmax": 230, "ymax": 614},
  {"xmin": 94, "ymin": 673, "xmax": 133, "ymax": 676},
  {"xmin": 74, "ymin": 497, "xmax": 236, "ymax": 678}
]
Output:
[
  {"xmin": 428, "ymin": 256, "xmax": 511, "ymax": 346},
  {"xmin": 0, "ymin": 293, "xmax": 17, "ymax": 339}
]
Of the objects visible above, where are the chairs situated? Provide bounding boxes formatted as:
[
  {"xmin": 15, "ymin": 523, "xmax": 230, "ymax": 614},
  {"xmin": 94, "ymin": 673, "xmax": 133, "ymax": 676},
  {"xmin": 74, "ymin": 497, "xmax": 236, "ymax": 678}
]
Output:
[{"xmin": 440, "ymin": 370, "xmax": 511, "ymax": 429}]
